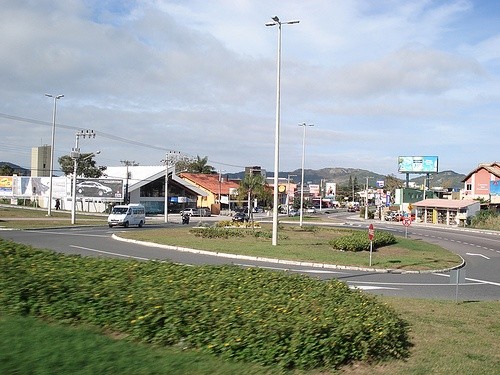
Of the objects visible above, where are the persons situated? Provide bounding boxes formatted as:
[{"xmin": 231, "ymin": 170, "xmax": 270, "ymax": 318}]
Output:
[{"xmin": 55, "ymin": 199, "xmax": 60, "ymax": 210}]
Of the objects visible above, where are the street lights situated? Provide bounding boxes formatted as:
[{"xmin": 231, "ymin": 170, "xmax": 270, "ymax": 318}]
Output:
[
  {"xmin": 264, "ymin": 15, "xmax": 300, "ymax": 245},
  {"xmin": 298, "ymin": 122, "xmax": 314, "ymax": 227},
  {"xmin": 71, "ymin": 150, "xmax": 101, "ymax": 224},
  {"xmin": 44, "ymin": 93, "xmax": 64, "ymax": 217}
]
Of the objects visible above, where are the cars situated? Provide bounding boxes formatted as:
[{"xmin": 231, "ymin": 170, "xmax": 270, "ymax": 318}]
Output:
[
  {"xmin": 180, "ymin": 208, "xmax": 212, "ymax": 217},
  {"xmin": 232, "ymin": 212, "xmax": 249, "ymax": 223},
  {"xmin": 385, "ymin": 212, "xmax": 415, "ymax": 222},
  {"xmin": 75, "ymin": 181, "xmax": 113, "ymax": 198},
  {"xmin": 347, "ymin": 205, "xmax": 360, "ymax": 212},
  {"xmin": 307, "ymin": 206, "xmax": 317, "ymax": 214}
]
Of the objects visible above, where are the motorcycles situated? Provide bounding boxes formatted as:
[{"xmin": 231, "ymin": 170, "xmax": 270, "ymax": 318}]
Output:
[{"xmin": 181, "ymin": 212, "xmax": 191, "ymax": 224}]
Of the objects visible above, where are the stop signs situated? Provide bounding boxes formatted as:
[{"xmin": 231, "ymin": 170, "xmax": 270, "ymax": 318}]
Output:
[{"xmin": 368, "ymin": 224, "xmax": 374, "ymax": 239}]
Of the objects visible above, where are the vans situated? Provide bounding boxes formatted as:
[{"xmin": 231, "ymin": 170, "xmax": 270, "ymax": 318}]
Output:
[{"xmin": 107, "ymin": 203, "xmax": 145, "ymax": 228}]
